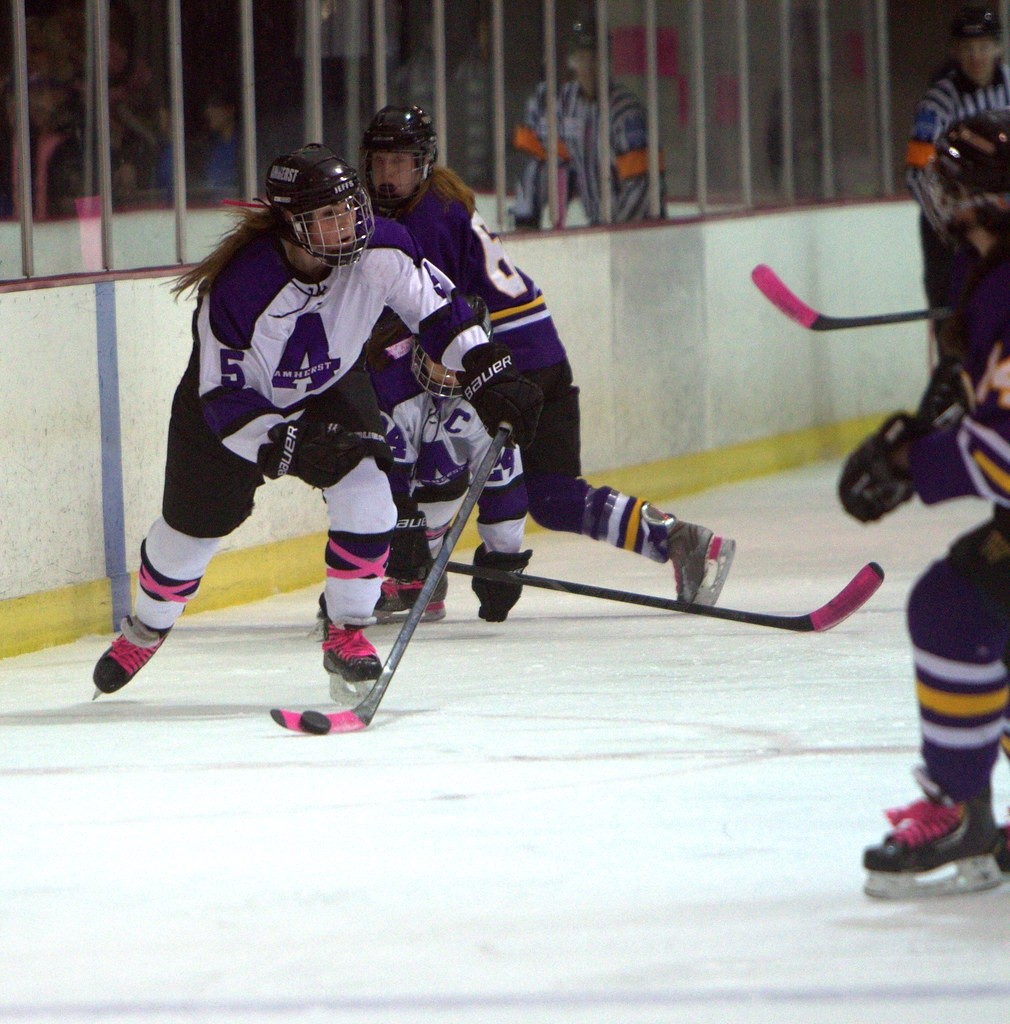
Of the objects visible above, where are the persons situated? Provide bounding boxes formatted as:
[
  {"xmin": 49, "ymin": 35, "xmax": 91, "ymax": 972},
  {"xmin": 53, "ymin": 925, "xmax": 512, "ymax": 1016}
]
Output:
[
  {"xmin": 0, "ymin": 72, "xmax": 284, "ymax": 216},
  {"xmin": 905, "ymin": 8, "xmax": 1010, "ymax": 334},
  {"xmin": 510, "ymin": 26, "xmax": 666, "ymax": 228},
  {"xmin": 311, "ymin": 295, "xmax": 533, "ymax": 641},
  {"xmin": 837, "ymin": 110, "xmax": 1010, "ymax": 898},
  {"xmin": 358, "ymin": 101, "xmax": 735, "ymax": 605},
  {"xmin": 93, "ymin": 142, "xmax": 544, "ymax": 707}
]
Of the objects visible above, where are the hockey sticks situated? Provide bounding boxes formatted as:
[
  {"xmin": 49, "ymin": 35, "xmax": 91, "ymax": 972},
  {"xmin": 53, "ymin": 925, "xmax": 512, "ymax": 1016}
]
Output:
[
  {"xmin": 418, "ymin": 559, "xmax": 888, "ymax": 634},
  {"xmin": 221, "ymin": 198, "xmax": 271, "ymax": 210},
  {"xmin": 267, "ymin": 420, "xmax": 514, "ymax": 733},
  {"xmin": 747, "ymin": 260, "xmax": 964, "ymax": 335}
]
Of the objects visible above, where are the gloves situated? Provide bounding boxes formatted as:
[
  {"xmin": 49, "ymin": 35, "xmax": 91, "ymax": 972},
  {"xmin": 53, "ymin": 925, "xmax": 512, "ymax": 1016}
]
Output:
[
  {"xmin": 913, "ymin": 357, "xmax": 976, "ymax": 437},
  {"xmin": 455, "ymin": 341, "xmax": 545, "ymax": 451},
  {"xmin": 387, "ymin": 497, "xmax": 433, "ymax": 583},
  {"xmin": 471, "ymin": 543, "xmax": 534, "ymax": 622},
  {"xmin": 839, "ymin": 410, "xmax": 916, "ymax": 522},
  {"xmin": 257, "ymin": 420, "xmax": 368, "ymax": 488}
]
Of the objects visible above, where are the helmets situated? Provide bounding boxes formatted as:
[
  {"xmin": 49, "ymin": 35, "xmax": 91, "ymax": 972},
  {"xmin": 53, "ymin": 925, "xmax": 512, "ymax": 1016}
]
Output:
[
  {"xmin": 360, "ymin": 105, "xmax": 438, "ymax": 207},
  {"xmin": 410, "ymin": 293, "xmax": 494, "ymax": 401},
  {"xmin": 914, "ymin": 106, "xmax": 1010, "ymax": 272},
  {"xmin": 252, "ymin": 143, "xmax": 375, "ymax": 267}
]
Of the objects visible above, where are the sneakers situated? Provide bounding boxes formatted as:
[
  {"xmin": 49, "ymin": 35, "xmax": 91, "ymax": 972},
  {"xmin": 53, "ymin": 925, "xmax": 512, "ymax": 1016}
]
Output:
[
  {"xmin": 666, "ymin": 513, "xmax": 737, "ymax": 607},
  {"xmin": 319, "ymin": 591, "xmax": 383, "ymax": 707},
  {"xmin": 92, "ymin": 613, "xmax": 174, "ymax": 702},
  {"xmin": 862, "ymin": 764, "xmax": 1009, "ymax": 898},
  {"xmin": 370, "ymin": 561, "xmax": 448, "ymax": 624}
]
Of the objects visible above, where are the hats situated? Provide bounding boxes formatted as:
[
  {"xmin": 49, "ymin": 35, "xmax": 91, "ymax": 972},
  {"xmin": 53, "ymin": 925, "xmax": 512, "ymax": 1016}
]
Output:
[
  {"xmin": 949, "ymin": 0, "xmax": 1003, "ymax": 39},
  {"xmin": 568, "ymin": 18, "xmax": 613, "ymax": 57}
]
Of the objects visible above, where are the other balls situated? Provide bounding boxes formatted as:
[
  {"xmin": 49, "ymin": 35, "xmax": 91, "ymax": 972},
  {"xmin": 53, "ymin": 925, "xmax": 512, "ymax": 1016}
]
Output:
[{"xmin": 300, "ymin": 711, "xmax": 332, "ymax": 735}]
